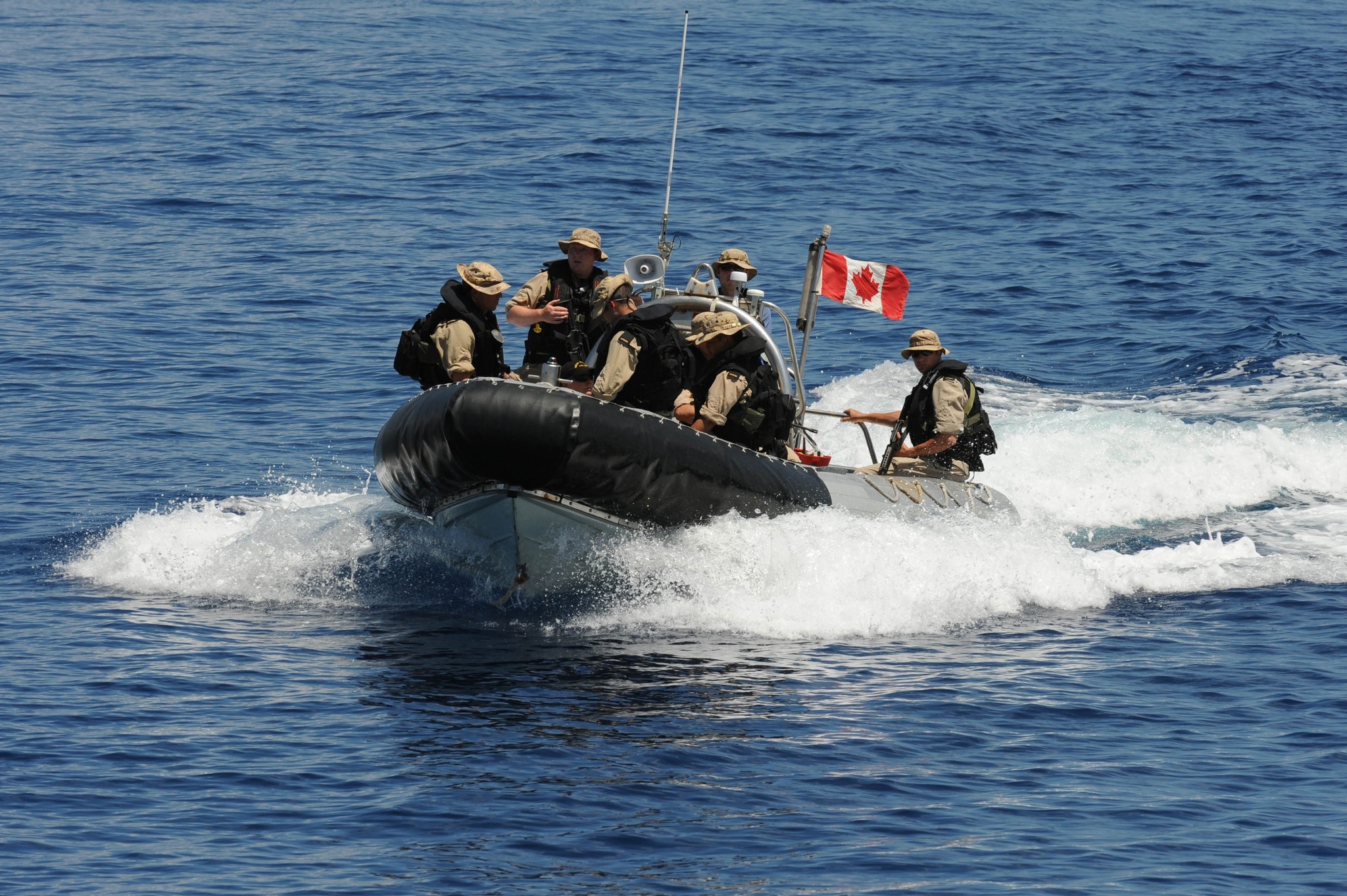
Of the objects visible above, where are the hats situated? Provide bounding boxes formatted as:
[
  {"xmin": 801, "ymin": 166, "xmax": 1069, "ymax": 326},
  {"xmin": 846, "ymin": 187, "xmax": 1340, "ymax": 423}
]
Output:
[
  {"xmin": 457, "ymin": 262, "xmax": 511, "ymax": 295},
  {"xmin": 558, "ymin": 227, "xmax": 608, "ymax": 262},
  {"xmin": 901, "ymin": 329, "xmax": 950, "ymax": 360},
  {"xmin": 590, "ymin": 274, "xmax": 633, "ymax": 320},
  {"xmin": 695, "ymin": 311, "xmax": 752, "ymax": 345},
  {"xmin": 685, "ymin": 311, "xmax": 715, "ymax": 342},
  {"xmin": 711, "ymin": 248, "xmax": 758, "ymax": 282}
]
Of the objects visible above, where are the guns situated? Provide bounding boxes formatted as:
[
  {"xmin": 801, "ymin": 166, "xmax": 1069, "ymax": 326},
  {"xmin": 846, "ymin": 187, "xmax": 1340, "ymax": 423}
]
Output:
[
  {"xmin": 475, "ymin": 330, "xmax": 511, "ymax": 375},
  {"xmin": 879, "ymin": 368, "xmax": 940, "ymax": 476},
  {"xmin": 790, "ymin": 422, "xmax": 818, "ymax": 434},
  {"xmin": 555, "ymin": 299, "xmax": 590, "ymax": 364}
]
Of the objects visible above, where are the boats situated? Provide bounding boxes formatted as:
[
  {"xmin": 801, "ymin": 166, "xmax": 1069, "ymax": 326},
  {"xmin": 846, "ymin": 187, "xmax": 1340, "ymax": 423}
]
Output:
[{"xmin": 373, "ymin": 10, "xmax": 1021, "ymax": 613}]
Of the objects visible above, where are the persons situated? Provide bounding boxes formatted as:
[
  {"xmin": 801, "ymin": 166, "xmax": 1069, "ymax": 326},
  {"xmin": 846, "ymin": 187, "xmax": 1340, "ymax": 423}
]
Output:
[
  {"xmin": 841, "ymin": 329, "xmax": 998, "ymax": 482},
  {"xmin": 673, "ymin": 310, "xmax": 797, "ymax": 461},
  {"xmin": 587, "ymin": 274, "xmax": 713, "ymax": 414},
  {"xmin": 393, "ymin": 261, "xmax": 524, "ymax": 391},
  {"xmin": 556, "ymin": 360, "xmax": 597, "ymax": 396},
  {"xmin": 691, "ymin": 247, "xmax": 771, "ymax": 342},
  {"xmin": 506, "ymin": 227, "xmax": 610, "ymax": 388}
]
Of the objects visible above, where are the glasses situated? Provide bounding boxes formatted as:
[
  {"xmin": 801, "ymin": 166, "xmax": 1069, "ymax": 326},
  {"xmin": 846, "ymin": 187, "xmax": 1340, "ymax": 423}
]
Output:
[{"xmin": 721, "ymin": 263, "xmax": 746, "ymax": 272}]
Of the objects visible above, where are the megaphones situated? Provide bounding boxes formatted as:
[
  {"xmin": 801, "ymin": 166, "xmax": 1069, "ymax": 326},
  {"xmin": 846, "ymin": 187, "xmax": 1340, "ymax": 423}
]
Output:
[{"xmin": 623, "ymin": 254, "xmax": 666, "ymax": 285}]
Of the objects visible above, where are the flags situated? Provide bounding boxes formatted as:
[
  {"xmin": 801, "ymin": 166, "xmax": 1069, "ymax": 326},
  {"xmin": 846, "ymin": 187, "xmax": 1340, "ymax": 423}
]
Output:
[{"xmin": 816, "ymin": 250, "xmax": 910, "ymax": 321}]
